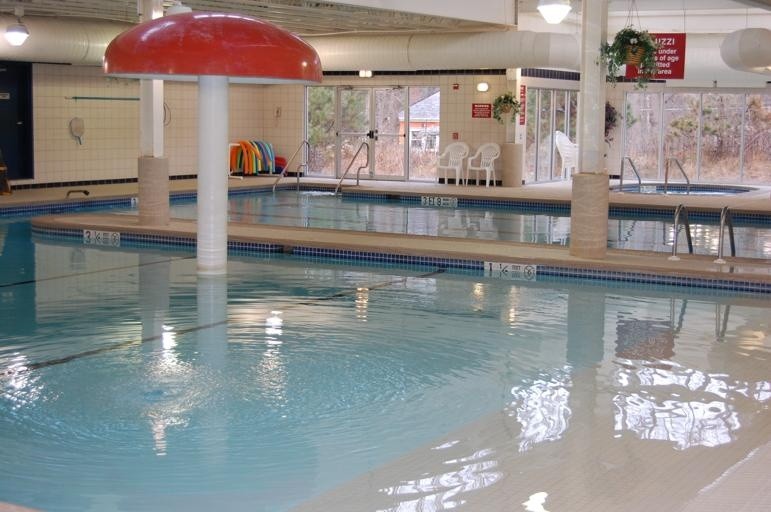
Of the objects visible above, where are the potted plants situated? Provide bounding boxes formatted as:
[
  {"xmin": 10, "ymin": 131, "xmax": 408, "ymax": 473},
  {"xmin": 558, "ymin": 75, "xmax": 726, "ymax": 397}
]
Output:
[
  {"xmin": 598, "ymin": 24, "xmax": 664, "ymax": 90},
  {"xmin": 492, "ymin": 92, "xmax": 521, "ymax": 125}
]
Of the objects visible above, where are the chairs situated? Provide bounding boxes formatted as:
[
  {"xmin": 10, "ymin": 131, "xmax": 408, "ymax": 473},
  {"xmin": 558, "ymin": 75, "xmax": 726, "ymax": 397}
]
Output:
[
  {"xmin": 465, "ymin": 142, "xmax": 501, "ymax": 189},
  {"xmin": 434, "ymin": 141, "xmax": 469, "ymax": 186},
  {"xmin": 556, "ymin": 131, "xmax": 578, "ymax": 185}
]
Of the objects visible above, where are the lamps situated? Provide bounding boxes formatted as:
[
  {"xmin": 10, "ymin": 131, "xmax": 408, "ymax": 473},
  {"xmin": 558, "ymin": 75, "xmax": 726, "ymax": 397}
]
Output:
[
  {"xmin": 536, "ymin": 0, "xmax": 572, "ymax": 24},
  {"xmin": 3, "ymin": 22, "xmax": 30, "ymax": 47},
  {"xmin": 477, "ymin": 83, "xmax": 489, "ymax": 92}
]
